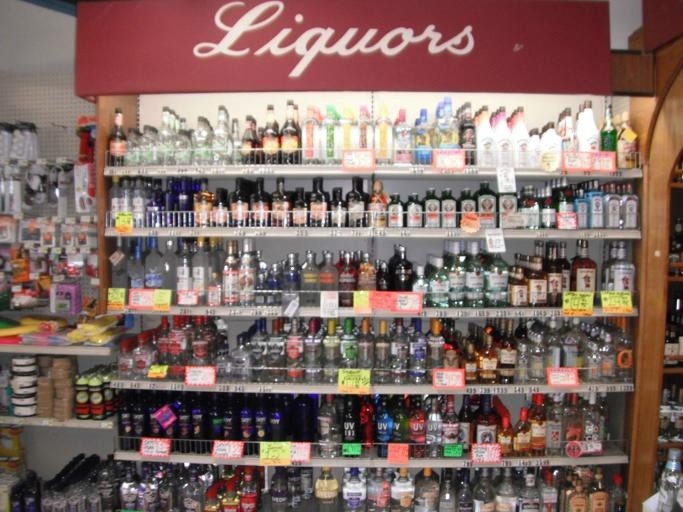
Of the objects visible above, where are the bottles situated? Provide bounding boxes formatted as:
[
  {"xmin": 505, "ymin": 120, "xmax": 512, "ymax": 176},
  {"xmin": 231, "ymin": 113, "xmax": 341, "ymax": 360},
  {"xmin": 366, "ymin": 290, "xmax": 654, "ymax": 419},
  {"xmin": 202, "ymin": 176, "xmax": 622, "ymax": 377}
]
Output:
[
  {"xmin": 658, "ymin": 382, "xmax": 683, "ymax": 446},
  {"xmin": 73, "ymin": 363, "xmax": 116, "ymax": 421},
  {"xmin": 115, "ymin": 391, "xmax": 610, "ymax": 459},
  {"xmin": 108, "ymin": 93, "xmax": 619, "ymax": 168},
  {"xmin": 10, "ymin": 467, "xmax": 40, "ymax": 512},
  {"xmin": 662, "ymin": 309, "xmax": 682, "ymax": 366},
  {"xmin": 116, "ymin": 315, "xmax": 632, "ymax": 386},
  {"xmin": 108, "ymin": 176, "xmax": 638, "ymax": 231},
  {"xmin": 40, "ymin": 446, "xmax": 116, "ymax": 512},
  {"xmin": 118, "ymin": 463, "xmax": 626, "ymax": 512},
  {"xmin": 667, "ymin": 216, "xmax": 682, "ymax": 275},
  {"xmin": 655, "ymin": 447, "xmax": 682, "ymax": 512},
  {"xmin": 106, "ymin": 235, "xmax": 637, "ymax": 305}
]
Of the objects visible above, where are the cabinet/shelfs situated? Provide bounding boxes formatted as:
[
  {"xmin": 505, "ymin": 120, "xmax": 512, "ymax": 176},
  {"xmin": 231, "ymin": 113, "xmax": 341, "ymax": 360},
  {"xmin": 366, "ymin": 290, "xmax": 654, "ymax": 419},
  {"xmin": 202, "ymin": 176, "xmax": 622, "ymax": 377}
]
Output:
[{"xmin": 2, "ymin": 44, "xmax": 683, "ymax": 512}]
